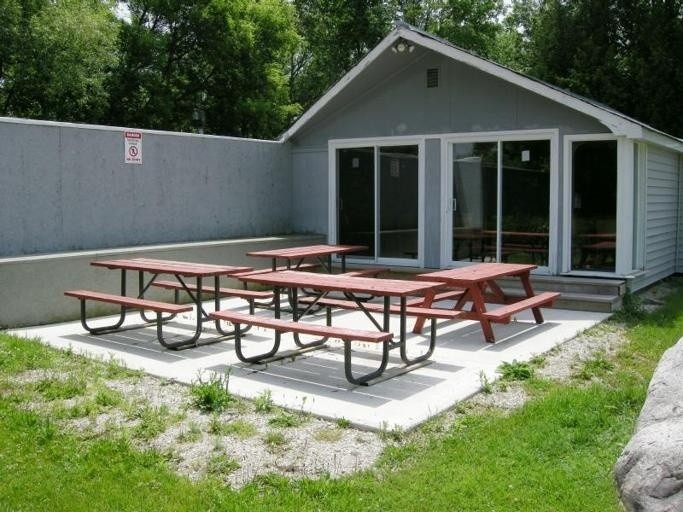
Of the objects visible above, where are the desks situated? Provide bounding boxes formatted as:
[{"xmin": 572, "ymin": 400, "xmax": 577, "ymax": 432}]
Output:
[
  {"xmin": 91, "ymin": 258, "xmax": 250, "ymax": 337},
  {"xmin": 448, "ymin": 228, "xmax": 615, "ymax": 251},
  {"xmin": 419, "ymin": 261, "xmax": 544, "ymax": 343},
  {"xmin": 240, "ymin": 269, "xmax": 445, "ymax": 365},
  {"xmin": 245, "ymin": 241, "xmax": 370, "ymax": 303}
]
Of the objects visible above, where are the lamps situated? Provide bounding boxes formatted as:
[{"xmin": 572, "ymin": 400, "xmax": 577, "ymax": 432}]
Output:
[{"xmin": 390, "ymin": 37, "xmax": 414, "ymax": 55}]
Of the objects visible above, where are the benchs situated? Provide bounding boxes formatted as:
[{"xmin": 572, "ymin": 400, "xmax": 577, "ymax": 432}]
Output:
[
  {"xmin": 393, "ymin": 286, "xmax": 465, "ymax": 309},
  {"xmin": 481, "ymin": 288, "xmax": 561, "ymax": 327},
  {"xmin": 468, "ymin": 242, "xmax": 547, "ymax": 264},
  {"xmin": 151, "ymin": 280, "xmax": 276, "ymax": 322},
  {"xmin": 335, "ymin": 266, "xmax": 391, "ymax": 281},
  {"xmin": 299, "ymin": 296, "xmax": 461, "ymax": 356},
  {"xmin": 61, "ymin": 288, "xmax": 197, "ymax": 348},
  {"xmin": 209, "ymin": 310, "xmax": 393, "ymax": 386},
  {"xmin": 225, "ymin": 260, "xmax": 316, "ymax": 284}
]
